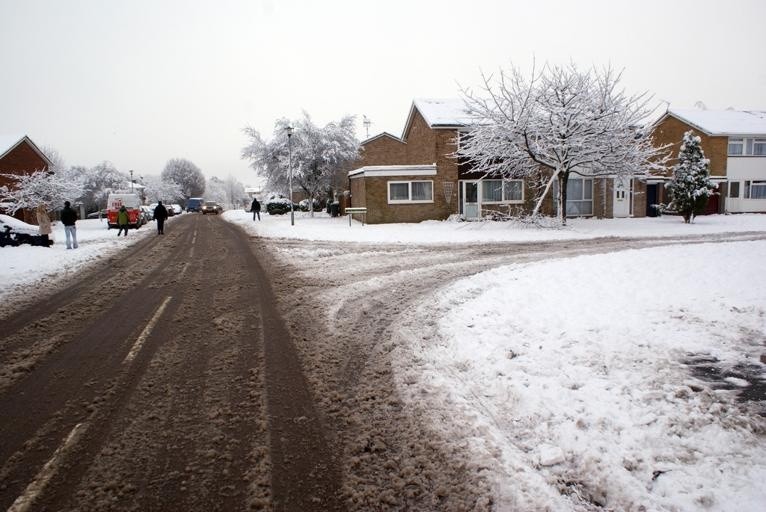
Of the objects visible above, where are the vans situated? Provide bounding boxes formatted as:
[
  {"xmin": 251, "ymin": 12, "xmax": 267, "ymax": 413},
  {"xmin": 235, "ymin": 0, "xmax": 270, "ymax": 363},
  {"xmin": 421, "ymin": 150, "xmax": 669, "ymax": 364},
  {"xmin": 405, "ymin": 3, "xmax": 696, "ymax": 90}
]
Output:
[
  {"xmin": 107, "ymin": 193, "xmax": 142, "ymax": 229},
  {"xmin": 186, "ymin": 198, "xmax": 204, "ymax": 213}
]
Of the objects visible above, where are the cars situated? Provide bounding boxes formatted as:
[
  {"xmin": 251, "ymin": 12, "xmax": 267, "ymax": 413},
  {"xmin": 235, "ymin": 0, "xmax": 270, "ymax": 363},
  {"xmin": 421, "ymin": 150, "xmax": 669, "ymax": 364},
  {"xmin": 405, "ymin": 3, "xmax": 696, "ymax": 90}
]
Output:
[
  {"xmin": 202, "ymin": 201, "xmax": 219, "ymax": 214},
  {"xmin": 143, "ymin": 204, "xmax": 183, "ymax": 220}
]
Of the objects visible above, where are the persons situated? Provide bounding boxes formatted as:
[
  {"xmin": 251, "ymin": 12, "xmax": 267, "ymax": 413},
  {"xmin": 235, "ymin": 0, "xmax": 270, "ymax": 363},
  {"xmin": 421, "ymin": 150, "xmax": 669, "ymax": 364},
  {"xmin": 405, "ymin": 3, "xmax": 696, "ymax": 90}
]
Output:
[
  {"xmin": 59, "ymin": 200, "xmax": 78, "ymax": 249},
  {"xmin": 152, "ymin": 200, "xmax": 168, "ymax": 235},
  {"xmin": 37, "ymin": 203, "xmax": 52, "ymax": 246},
  {"xmin": 250, "ymin": 197, "xmax": 261, "ymax": 221},
  {"xmin": 117, "ymin": 204, "xmax": 130, "ymax": 237}
]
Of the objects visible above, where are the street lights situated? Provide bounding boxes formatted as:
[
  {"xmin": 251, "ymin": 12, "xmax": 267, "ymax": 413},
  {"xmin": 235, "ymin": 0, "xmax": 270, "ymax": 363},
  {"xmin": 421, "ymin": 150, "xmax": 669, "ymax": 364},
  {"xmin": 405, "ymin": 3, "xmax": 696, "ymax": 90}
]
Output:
[{"xmin": 283, "ymin": 125, "xmax": 295, "ymax": 225}]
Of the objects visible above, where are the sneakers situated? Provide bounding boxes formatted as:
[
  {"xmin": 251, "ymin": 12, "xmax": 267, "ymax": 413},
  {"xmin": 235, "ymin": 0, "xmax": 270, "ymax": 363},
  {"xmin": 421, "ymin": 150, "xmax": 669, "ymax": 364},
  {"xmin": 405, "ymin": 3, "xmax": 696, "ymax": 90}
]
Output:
[
  {"xmin": 66, "ymin": 245, "xmax": 78, "ymax": 249},
  {"xmin": 158, "ymin": 231, "xmax": 164, "ymax": 235}
]
